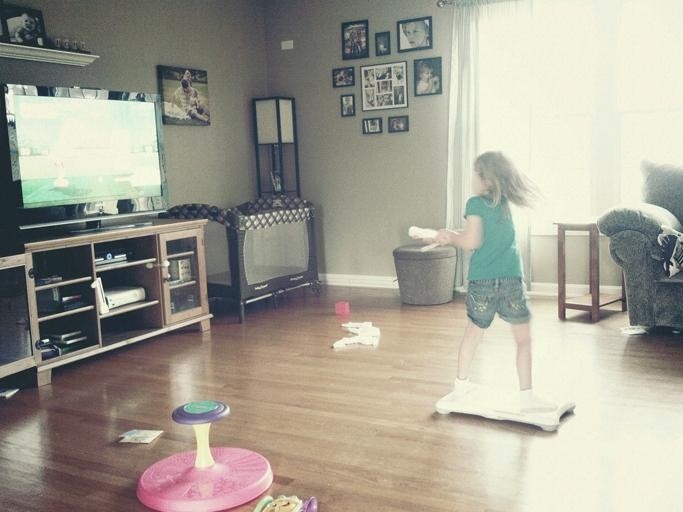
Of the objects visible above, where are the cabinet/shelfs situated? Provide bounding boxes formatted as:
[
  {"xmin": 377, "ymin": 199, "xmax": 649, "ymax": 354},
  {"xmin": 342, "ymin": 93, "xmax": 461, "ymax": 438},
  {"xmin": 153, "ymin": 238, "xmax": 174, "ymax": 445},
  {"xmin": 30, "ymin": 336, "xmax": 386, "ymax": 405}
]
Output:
[{"xmin": 0, "ymin": 217, "xmax": 215, "ymax": 392}]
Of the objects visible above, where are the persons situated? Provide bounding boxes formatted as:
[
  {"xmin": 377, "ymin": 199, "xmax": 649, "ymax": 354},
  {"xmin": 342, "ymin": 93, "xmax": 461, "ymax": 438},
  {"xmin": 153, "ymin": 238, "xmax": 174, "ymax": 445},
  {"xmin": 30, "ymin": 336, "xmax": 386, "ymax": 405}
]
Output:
[
  {"xmin": 379, "ymin": 36, "xmax": 384, "ymax": 54},
  {"xmin": 184, "ymin": 70, "xmax": 192, "ymax": 87},
  {"xmin": 356, "ymin": 29, "xmax": 363, "ymax": 53},
  {"xmin": 392, "ymin": 119, "xmax": 399, "ymax": 130},
  {"xmin": 349, "ymin": 29, "xmax": 357, "ymax": 53},
  {"xmin": 336, "ymin": 71, "xmax": 344, "ymax": 85},
  {"xmin": 425, "ymin": 153, "xmax": 557, "ymax": 413},
  {"xmin": 402, "ymin": 20, "xmax": 430, "ymax": 48},
  {"xmin": 171, "ymin": 80, "xmax": 211, "ymax": 123},
  {"xmin": 366, "ymin": 68, "xmax": 403, "ymax": 106},
  {"xmin": 343, "ymin": 98, "xmax": 352, "ymax": 115},
  {"xmin": 416, "ymin": 61, "xmax": 440, "ymax": 94},
  {"xmin": 15, "ymin": 13, "xmax": 43, "ymax": 46}
]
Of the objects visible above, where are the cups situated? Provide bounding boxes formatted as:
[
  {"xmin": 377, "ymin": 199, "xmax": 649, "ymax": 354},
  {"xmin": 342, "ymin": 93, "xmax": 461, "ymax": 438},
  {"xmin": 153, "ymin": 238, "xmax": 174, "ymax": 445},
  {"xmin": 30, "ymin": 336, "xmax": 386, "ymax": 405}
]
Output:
[
  {"xmin": 335, "ymin": 301, "xmax": 350, "ymax": 315},
  {"xmin": 54, "ymin": 37, "xmax": 87, "ymax": 52}
]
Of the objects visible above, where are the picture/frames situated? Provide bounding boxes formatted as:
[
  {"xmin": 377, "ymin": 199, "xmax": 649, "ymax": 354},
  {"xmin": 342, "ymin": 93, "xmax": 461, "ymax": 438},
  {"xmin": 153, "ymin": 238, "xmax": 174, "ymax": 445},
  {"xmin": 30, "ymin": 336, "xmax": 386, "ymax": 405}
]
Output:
[
  {"xmin": 327, "ymin": 54, "xmax": 446, "ymax": 137},
  {"xmin": 395, "ymin": 16, "xmax": 433, "ymax": 54},
  {"xmin": 0, "ymin": 4, "xmax": 51, "ymax": 49},
  {"xmin": 338, "ymin": 16, "xmax": 370, "ymax": 61},
  {"xmin": 373, "ymin": 31, "xmax": 391, "ymax": 58}
]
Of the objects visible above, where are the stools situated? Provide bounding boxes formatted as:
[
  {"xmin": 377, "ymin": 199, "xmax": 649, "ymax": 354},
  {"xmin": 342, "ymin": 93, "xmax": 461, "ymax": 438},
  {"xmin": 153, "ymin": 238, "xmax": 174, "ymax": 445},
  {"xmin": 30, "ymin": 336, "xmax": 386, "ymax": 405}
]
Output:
[{"xmin": 393, "ymin": 243, "xmax": 459, "ymax": 310}]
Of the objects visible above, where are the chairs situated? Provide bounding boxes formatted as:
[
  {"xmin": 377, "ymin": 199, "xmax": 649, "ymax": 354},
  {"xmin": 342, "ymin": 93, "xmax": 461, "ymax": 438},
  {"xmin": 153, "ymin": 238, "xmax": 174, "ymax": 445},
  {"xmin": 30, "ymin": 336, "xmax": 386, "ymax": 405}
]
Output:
[{"xmin": 592, "ymin": 157, "xmax": 683, "ymax": 338}]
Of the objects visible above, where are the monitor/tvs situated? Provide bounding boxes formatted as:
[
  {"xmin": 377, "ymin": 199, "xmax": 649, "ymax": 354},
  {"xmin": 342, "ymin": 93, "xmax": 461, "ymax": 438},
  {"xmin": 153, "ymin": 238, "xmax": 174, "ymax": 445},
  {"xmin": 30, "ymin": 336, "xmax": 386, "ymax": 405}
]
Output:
[{"xmin": 0, "ymin": 84, "xmax": 168, "ymax": 234}]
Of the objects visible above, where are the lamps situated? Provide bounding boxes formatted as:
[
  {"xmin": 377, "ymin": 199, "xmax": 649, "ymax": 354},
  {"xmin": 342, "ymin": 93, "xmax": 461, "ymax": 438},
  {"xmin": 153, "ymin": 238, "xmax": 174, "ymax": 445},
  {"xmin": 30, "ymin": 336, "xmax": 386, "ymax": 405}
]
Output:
[{"xmin": 250, "ymin": 92, "xmax": 302, "ymax": 200}]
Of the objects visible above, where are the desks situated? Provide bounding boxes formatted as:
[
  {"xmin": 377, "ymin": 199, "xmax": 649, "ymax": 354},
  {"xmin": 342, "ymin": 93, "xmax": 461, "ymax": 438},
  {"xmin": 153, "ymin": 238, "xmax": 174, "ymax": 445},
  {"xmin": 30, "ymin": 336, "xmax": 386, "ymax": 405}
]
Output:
[{"xmin": 550, "ymin": 213, "xmax": 630, "ymax": 325}]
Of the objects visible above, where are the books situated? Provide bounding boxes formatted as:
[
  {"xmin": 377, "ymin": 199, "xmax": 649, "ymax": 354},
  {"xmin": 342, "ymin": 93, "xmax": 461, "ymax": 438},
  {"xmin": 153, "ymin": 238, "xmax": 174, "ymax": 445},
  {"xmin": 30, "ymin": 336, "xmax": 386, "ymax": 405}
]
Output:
[
  {"xmin": 48, "ymin": 329, "xmax": 90, "ymax": 355},
  {"xmin": 54, "ymin": 295, "xmax": 84, "ymax": 311},
  {"xmin": 104, "ymin": 285, "xmax": 146, "ymax": 310}
]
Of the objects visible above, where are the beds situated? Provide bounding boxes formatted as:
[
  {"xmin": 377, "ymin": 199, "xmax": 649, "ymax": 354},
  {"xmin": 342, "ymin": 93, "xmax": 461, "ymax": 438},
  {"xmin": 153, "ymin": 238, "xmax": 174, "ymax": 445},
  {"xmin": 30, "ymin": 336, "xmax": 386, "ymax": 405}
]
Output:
[{"xmin": 153, "ymin": 194, "xmax": 324, "ymax": 326}]
